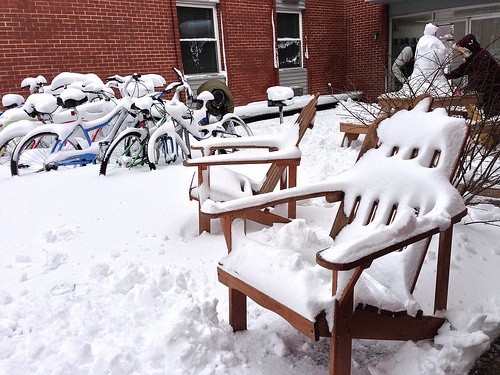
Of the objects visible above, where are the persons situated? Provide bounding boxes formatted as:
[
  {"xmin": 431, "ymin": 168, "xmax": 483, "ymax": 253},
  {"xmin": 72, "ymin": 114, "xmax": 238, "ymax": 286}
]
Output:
[
  {"xmin": 392, "ymin": 23, "xmax": 500, "ymax": 120},
  {"xmin": 196, "ymin": 79, "xmax": 240, "ymax": 157}
]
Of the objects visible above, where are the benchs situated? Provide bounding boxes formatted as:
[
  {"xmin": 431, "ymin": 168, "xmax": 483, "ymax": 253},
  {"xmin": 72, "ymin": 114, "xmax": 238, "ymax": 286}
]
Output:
[{"xmin": 338, "ymin": 121, "xmax": 370, "ymax": 148}]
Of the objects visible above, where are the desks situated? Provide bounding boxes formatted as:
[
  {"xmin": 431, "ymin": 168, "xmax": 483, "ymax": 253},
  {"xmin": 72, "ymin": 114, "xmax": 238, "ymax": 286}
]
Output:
[{"xmin": 376, "ymin": 93, "xmax": 483, "ymax": 119}]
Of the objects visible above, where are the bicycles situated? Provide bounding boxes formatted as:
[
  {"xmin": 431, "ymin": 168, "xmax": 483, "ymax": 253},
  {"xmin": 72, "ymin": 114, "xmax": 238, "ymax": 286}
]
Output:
[{"xmin": 0, "ymin": 70, "xmax": 254, "ymax": 177}]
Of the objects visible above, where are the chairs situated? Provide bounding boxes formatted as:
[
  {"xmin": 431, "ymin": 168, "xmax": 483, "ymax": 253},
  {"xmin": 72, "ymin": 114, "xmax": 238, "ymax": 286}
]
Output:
[
  {"xmin": 182, "ymin": 91, "xmax": 322, "ymax": 239},
  {"xmin": 200, "ymin": 119, "xmax": 472, "ymax": 375}
]
